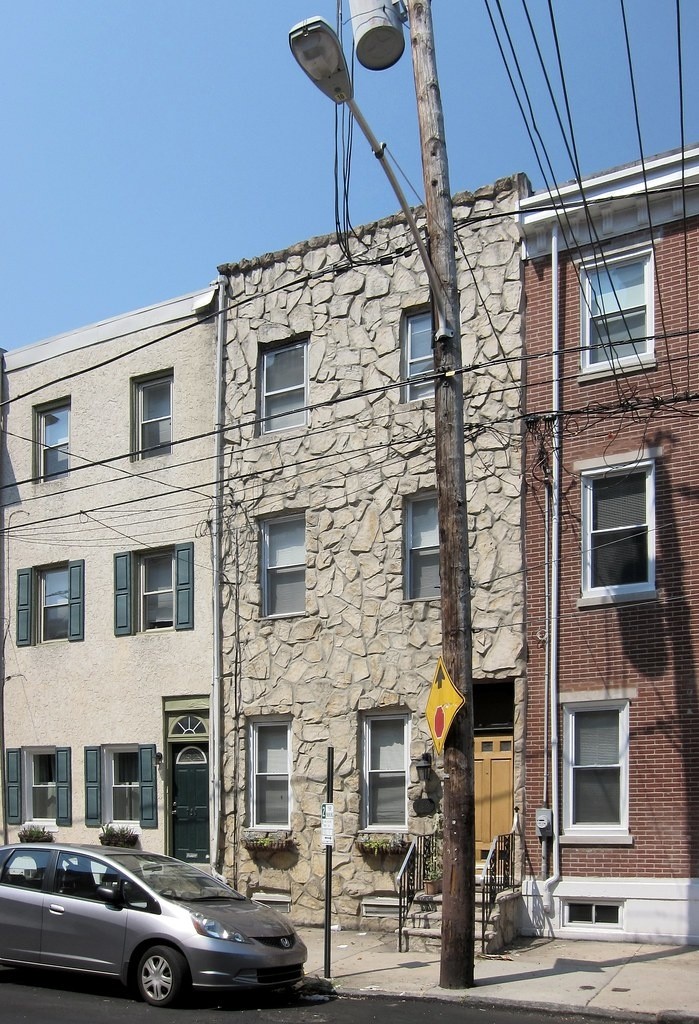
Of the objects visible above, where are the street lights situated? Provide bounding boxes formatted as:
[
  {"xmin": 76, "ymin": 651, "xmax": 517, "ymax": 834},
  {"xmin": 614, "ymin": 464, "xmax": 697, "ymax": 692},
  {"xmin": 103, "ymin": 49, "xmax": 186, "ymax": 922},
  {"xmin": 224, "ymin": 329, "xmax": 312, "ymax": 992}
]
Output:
[{"xmin": 287, "ymin": 16, "xmax": 476, "ymax": 989}]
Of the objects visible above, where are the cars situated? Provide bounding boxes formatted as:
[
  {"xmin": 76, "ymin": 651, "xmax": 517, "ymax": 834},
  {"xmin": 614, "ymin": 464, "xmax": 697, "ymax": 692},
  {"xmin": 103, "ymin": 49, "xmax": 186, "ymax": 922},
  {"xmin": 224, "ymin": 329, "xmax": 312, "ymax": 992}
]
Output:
[{"xmin": 0, "ymin": 841, "xmax": 308, "ymax": 1006}]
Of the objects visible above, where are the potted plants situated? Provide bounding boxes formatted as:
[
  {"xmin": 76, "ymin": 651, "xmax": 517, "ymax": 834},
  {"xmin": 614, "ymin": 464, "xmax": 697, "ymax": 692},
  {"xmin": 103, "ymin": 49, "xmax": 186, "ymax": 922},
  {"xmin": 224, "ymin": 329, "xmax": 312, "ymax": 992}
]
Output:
[
  {"xmin": 355, "ymin": 837, "xmax": 408, "ymax": 855},
  {"xmin": 19, "ymin": 824, "xmax": 53, "ymax": 843},
  {"xmin": 99, "ymin": 822, "xmax": 140, "ymax": 848},
  {"xmin": 424, "ymin": 849, "xmax": 443, "ymax": 894},
  {"xmin": 241, "ymin": 834, "xmax": 297, "ymax": 850}
]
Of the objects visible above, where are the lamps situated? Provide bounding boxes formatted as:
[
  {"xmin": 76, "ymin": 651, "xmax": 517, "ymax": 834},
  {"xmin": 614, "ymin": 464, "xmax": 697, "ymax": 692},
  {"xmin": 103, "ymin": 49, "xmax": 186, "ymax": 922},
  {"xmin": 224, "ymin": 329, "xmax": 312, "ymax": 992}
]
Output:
[
  {"xmin": 416, "ymin": 753, "xmax": 432, "ymax": 781},
  {"xmin": 151, "ymin": 752, "xmax": 162, "ymax": 766}
]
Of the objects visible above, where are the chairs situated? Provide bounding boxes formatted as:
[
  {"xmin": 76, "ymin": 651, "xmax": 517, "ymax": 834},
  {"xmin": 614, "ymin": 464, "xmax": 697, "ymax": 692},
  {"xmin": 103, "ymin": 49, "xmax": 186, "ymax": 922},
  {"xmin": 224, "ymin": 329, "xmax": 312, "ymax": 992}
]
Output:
[
  {"xmin": 6, "ymin": 874, "xmax": 32, "ymax": 887},
  {"xmin": 34, "ymin": 864, "xmax": 101, "ymax": 899},
  {"xmin": 102, "ymin": 867, "xmax": 140, "ymax": 897}
]
